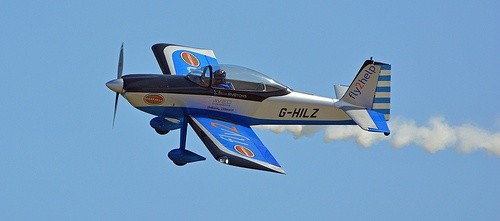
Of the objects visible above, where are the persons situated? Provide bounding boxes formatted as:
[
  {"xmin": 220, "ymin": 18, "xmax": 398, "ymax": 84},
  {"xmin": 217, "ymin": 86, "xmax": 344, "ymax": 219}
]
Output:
[{"xmin": 202, "ymin": 69, "xmax": 235, "ymax": 91}]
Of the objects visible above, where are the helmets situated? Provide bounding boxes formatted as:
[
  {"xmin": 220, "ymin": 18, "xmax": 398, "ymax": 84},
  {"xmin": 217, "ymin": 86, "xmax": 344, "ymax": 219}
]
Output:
[{"xmin": 213, "ymin": 70, "xmax": 227, "ymax": 83}]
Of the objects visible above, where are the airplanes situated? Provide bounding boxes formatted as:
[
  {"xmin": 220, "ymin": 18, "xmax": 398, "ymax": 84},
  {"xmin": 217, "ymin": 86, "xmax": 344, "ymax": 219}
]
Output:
[{"xmin": 105, "ymin": 42, "xmax": 392, "ymax": 174}]
